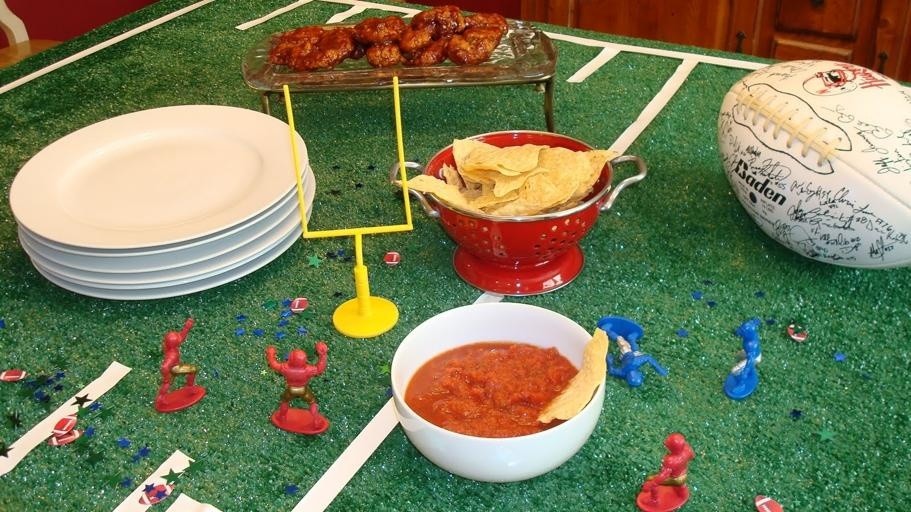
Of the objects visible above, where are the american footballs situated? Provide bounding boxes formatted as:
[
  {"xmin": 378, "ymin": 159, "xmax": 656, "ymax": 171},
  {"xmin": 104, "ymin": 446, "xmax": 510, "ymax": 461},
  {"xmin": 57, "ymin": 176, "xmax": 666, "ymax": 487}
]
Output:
[{"xmin": 718, "ymin": 59, "xmax": 911, "ymax": 267}]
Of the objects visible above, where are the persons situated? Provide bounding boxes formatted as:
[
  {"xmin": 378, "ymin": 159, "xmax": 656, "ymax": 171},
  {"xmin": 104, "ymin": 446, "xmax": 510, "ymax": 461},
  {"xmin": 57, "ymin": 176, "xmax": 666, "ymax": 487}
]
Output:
[
  {"xmin": 635, "ymin": 432, "xmax": 697, "ymax": 512},
  {"xmin": 596, "ymin": 316, "xmax": 667, "ymax": 388},
  {"xmin": 723, "ymin": 317, "xmax": 763, "ymax": 398},
  {"xmin": 266, "ymin": 341, "xmax": 330, "ymax": 435},
  {"xmin": 155, "ymin": 318, "xmax": 206, "ymax": 412}
]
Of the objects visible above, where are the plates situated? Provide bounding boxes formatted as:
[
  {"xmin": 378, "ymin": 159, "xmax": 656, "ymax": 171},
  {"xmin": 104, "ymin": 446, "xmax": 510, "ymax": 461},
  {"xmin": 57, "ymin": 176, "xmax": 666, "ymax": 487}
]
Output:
[
  {"xmin": 241, "ymin": 18, "xmax": 560, "ymax": 97},
  {"xmin": 3, "ymin": 100, "xmax": 322, "ymax": 302}
]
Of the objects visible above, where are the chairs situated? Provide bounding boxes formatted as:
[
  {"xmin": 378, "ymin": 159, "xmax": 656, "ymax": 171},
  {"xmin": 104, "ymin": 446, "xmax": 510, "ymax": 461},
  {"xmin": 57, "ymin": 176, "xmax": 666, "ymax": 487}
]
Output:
[{"xmin": 0, "ymin": 0, "xmax": 64, "ymax": 70}]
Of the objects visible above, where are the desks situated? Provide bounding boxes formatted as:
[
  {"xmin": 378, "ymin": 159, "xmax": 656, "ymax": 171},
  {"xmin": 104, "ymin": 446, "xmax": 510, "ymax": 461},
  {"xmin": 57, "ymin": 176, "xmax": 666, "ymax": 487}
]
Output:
[{"xmin": 1, "ymin": 0, "xmax": 910, "ymax": 512}]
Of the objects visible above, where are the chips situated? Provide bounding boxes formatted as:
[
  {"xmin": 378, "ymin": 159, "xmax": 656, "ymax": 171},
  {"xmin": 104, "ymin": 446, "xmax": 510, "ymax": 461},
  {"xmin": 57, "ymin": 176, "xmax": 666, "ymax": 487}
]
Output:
[
  {"xmin": 395, "ymin": 134, "xmax": 619, "ymax": 217},
  {"xmin": 537, "ymin": 328, "xmax": 610, "ymax": 425}
]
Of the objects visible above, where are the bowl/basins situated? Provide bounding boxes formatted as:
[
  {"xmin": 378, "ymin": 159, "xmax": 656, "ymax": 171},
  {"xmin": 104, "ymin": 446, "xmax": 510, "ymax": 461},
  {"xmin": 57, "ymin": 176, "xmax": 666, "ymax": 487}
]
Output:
[{"xmin": 389, "ymin": 297, "xmax": 609, "ymax": 484}]
